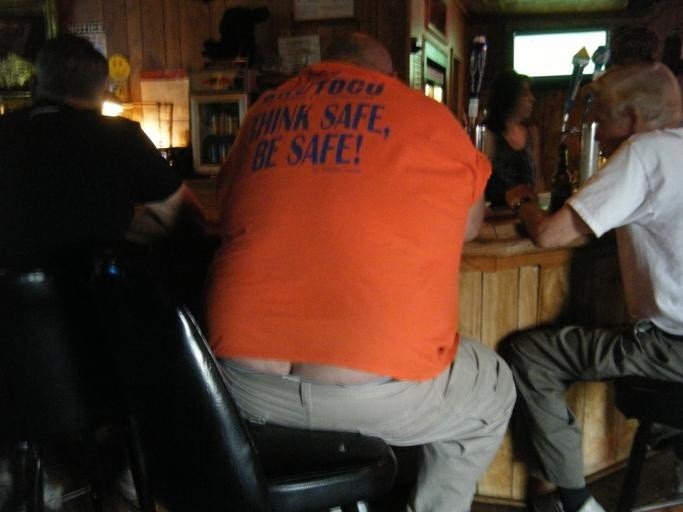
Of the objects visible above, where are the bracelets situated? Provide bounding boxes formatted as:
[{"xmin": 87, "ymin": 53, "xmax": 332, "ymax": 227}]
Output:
[{"xmin": 511, "ymin": 192, "xmax": 537, "ymax": 212}]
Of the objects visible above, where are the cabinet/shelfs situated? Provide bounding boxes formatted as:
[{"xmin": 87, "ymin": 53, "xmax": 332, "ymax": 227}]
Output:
[{"xmin": 190, "ymin": 90, "xmax": 250, "ymax": 178}]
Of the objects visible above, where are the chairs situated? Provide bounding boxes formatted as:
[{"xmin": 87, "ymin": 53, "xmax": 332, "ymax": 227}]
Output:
[
  {"xmin": 2, "ymin": 257, "xmax": 181, "ymax": 508},
  {"xmin": 135, "ymin": 274, "xmax": 396, "ymax": 511}
]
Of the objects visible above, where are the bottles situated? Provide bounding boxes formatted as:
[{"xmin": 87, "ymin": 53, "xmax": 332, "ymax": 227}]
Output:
[{"xmin": 549, "ymin": 143, "xmax": 574, "ymax": 214}]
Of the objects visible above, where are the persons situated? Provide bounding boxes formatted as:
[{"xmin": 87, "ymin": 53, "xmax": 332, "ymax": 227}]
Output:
[
  {"xmin": 495, "ymin": 61, "xmax": 683, "ymax": 510},
  {"xmin": 0, "ymin": 32, "xmax": 209, "ymax": 511},
  {"xmin": 469, "ymin": 72, "xmax": 545, "ymax": 209},
  {"xmin": 197, "ymin": 32, "xmax": 517, "ymax": 511}
]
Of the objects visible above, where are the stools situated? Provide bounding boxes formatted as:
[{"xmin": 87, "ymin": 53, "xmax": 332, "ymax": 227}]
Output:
[{"xmin": 614, "ymin": 375, "xmax": 683, "ymax": 512}]
